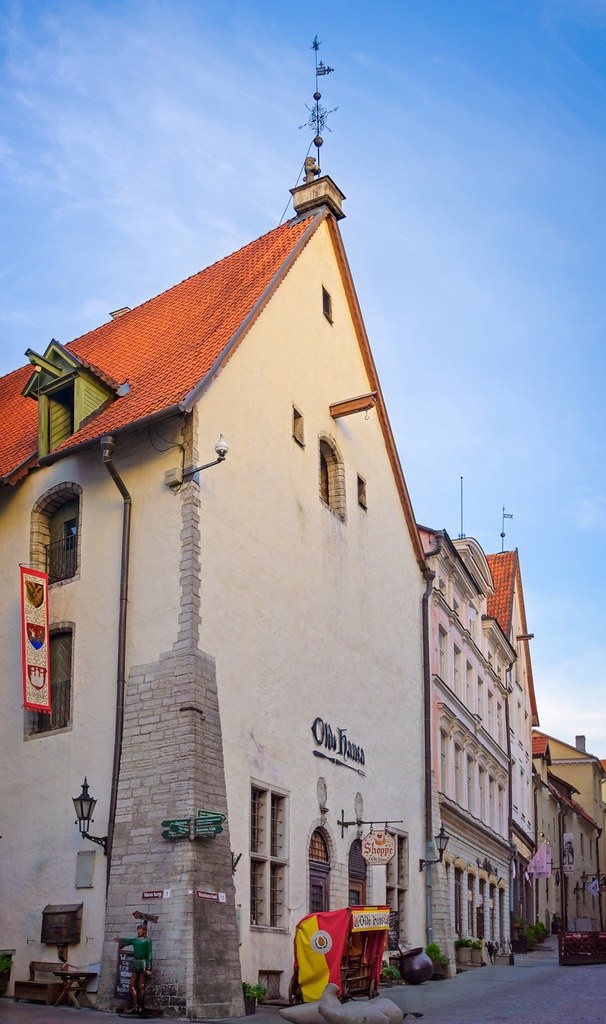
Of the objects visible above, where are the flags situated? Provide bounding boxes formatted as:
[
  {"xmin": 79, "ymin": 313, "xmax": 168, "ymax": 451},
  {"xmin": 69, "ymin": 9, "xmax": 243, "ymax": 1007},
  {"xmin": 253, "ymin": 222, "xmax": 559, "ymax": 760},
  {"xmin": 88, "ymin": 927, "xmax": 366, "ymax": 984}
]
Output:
[
  {"xmin": 587, "ymin": 879, "xmax": 599, "ymax": 896},
  {"xmin": 527, "ymin": 841, "xmax": 551, "ymax": 878}
]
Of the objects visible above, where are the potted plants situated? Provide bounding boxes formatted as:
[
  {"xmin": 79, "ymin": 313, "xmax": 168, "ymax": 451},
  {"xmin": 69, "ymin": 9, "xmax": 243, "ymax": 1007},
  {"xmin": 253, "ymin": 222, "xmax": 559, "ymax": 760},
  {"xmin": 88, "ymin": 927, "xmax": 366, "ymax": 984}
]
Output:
[
  {"xmin": 0, "ymin": 953, "xmax": 14, "ymax": 999},
  {"xmin": 470, "ymin": 940, "xmax": 484, "ymax": 963},
  {"xmin": 242, "ymin": 982, "xmax": 268, "ymax": 1014},
  {"xmin": 381, "ymin": 966, "xmax": 401, "ymax": 983},
  {"xmin": 455, "ymin": 939, "xmax": 473, "ymax": 963},
  {"xmin": 388, "ymin": 912, "xmax": 435, "ymax": 984}
]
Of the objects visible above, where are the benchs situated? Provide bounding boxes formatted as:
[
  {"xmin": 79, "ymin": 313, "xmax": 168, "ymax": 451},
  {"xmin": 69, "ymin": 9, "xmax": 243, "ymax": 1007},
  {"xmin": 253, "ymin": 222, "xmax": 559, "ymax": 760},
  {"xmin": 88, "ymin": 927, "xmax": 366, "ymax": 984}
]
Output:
[{"xmin": 14, "ymin": 960, "xmax": 69, "ymax": 1005}]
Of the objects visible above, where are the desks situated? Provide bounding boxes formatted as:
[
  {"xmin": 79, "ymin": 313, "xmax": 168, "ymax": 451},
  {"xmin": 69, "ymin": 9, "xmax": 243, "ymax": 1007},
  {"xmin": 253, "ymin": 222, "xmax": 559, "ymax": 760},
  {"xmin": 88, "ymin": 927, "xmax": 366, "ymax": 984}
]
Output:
[{"xmin": 52, "ymin": 971, "xmax": 98, "ymax": 1009}]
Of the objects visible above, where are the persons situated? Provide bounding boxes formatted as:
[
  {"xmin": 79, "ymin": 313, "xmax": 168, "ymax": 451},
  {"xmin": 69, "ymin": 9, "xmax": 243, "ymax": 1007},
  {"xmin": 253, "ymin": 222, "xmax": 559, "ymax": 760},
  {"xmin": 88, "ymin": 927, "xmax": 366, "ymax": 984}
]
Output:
[{"xmin": 113, "ymin": 925, "xmax": 152, "ymax": 1013}]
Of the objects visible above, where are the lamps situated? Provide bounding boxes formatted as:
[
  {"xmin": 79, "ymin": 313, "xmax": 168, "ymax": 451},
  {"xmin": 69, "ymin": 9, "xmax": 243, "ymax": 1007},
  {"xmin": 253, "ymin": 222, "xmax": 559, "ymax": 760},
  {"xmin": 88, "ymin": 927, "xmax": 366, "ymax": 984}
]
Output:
[
  {"xmin": 71, "ymin": 776, "xmax": 107, "ymax": 857},
  {"xmin": 419, "ymin": 820, "xmax": 451, "ymax": 872}
]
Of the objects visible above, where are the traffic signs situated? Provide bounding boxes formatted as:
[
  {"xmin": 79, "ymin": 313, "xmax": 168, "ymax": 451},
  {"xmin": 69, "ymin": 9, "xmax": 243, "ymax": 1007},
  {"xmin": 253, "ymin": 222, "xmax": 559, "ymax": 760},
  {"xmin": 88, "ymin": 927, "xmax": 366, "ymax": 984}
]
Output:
[{"xmin": 161, "ymin": 809, "xmax": 224, "ymax": 840}]
